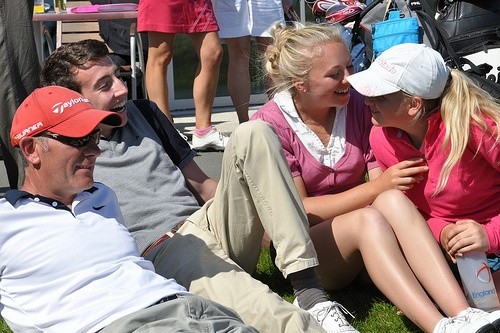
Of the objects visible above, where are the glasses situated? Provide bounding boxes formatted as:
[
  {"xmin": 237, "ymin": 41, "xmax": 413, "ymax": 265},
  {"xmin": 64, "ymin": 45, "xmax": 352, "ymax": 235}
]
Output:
[{"xmin": 33, "ymin": 129, "xmax": 101, "ymax": 148}]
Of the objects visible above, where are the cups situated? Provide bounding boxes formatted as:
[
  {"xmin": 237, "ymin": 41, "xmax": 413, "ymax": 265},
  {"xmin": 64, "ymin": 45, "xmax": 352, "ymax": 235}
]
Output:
[
  {"xmin": 54, "ymin": 0, "xmax": 67, "ymax": 13},
  {"xmin": 33, "ymin": 0, "xmax": 44, "ymax": 13}
]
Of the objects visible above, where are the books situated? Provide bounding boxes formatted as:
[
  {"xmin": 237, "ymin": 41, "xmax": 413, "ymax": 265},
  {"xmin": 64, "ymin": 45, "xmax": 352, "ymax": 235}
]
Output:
[{"xmin": 72, "ymin": 3, "xmax": 139, "ymax": 12}]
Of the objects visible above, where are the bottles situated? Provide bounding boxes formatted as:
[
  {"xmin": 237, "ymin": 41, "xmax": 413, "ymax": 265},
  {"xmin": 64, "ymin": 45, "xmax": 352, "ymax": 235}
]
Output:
[{"xmin": 456, "ymin": 251, "xmax": 500, "ymax": 311}]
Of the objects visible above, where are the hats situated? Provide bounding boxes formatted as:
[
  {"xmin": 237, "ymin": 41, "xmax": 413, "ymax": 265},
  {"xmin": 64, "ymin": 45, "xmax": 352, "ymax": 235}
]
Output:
[
  {"xmin": 10, "ymin": 86, "xmax": 123, "ymax": 148},
  {"xmin": 346, "ymin": 44, "xmax": 449, "ymax": 100}
]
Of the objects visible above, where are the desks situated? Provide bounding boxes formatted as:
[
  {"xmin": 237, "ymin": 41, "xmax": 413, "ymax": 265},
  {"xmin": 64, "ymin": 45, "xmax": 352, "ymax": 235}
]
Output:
[{"xmin": 33, "ymin": 12, "xmax": 148, "ymax": 100}]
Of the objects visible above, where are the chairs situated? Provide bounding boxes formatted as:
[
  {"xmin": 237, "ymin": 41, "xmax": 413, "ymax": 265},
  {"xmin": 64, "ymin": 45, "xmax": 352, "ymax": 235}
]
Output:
[{"xmin": 56, "ymin": 1, "xmax": 143, "ymax": 79}]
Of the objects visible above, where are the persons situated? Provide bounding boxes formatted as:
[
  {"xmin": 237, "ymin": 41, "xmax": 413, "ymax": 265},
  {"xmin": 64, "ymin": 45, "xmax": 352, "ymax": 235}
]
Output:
[
  {"xmin": 0, "ymin": 0, "xmax": 500, "ymax": 333},
  {"xmin": 247, "ymin": 22, "xmax": 500, "ymax": 333}
]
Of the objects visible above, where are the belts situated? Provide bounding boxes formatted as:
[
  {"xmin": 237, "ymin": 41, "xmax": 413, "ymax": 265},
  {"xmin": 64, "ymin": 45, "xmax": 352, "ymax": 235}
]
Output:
[{"xmin": 141, "ymin": 219, "xmax": 186, "ymax": 257}]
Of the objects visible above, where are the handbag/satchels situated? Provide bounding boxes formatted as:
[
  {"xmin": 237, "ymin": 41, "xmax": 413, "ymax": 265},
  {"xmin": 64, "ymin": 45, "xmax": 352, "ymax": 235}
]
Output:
[{"xmin": 360, "ymin": 0, "xmax": 439, "ymax": 61}]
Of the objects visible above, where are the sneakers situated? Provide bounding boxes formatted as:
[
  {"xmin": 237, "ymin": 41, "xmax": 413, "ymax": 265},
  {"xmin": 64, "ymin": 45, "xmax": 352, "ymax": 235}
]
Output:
[
  {"xmin": 293, "ymin": 296, "xmax": 360, "ymax": 333},
  {"xmin": 433, "ymin": 317, "xmax": 496, "ymax": 333},
  {"xmin": 176, "ymin": 128, "xmax": 194, "ymax": 150},
  {"xmin": 456, "ymin": 306, "xmax": 500, "ymax": 331},
  {"xmin": 192, "ymin": 127, "xmax": 229, "ymax": 151}
]
